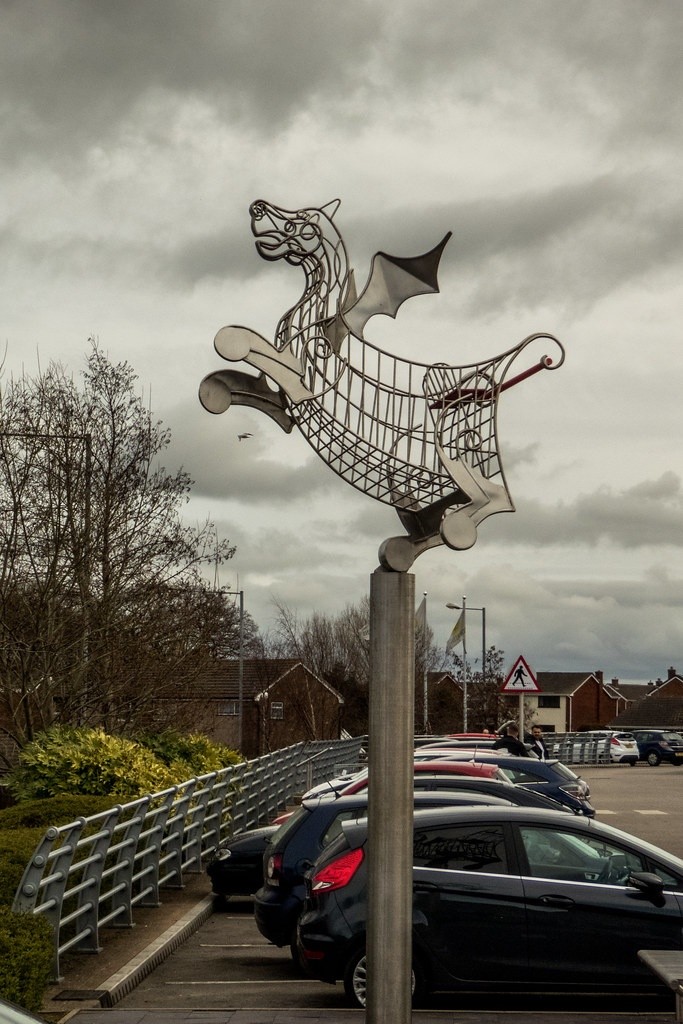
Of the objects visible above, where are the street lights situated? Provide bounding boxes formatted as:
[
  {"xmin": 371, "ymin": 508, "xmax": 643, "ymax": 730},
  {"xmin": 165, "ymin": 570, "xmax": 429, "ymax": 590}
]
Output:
[{"xmin": 445, "ymin": 602, "xmax": 486, "ymax": 682}]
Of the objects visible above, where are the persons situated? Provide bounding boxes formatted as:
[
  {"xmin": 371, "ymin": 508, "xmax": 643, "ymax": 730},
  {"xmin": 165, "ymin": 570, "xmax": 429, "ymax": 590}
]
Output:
[
  {"xmin": 525, "ymin": 726, "xmax": 549, "ymax": 759},
  {"xmin": 483, "ymin": 729, "xmax": 489, "ymax": 734},
  {"xmin": 491, "ymin": 722, "xmax": 532, "ymax": 757}
]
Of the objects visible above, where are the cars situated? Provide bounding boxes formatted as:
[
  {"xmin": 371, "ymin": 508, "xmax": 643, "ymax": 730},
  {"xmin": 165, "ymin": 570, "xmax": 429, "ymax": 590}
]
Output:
[
  {"xmin": 631, "ymin": 729, "xmax": 683, "ymax": 766},
  {"xmin": 206, "ymin": 732, "xmax": 682, "ymax": 1008},
  {"xmin": 552, "ymin": 730, "xmax": 640, "ymax": 766}
]
